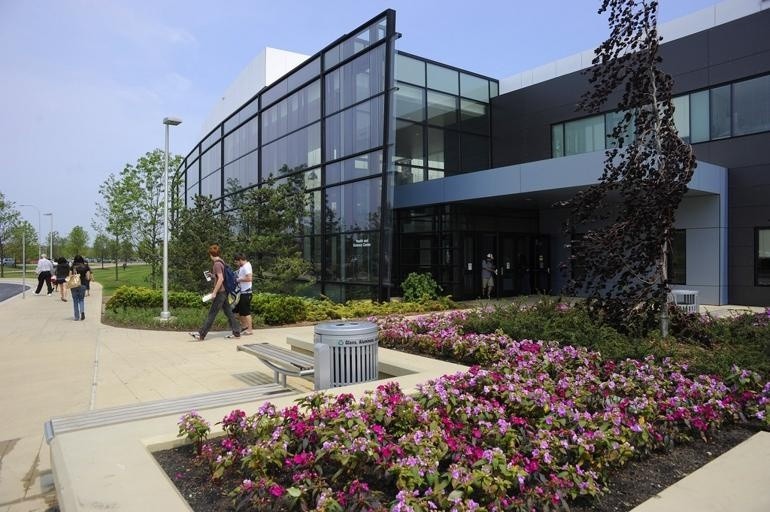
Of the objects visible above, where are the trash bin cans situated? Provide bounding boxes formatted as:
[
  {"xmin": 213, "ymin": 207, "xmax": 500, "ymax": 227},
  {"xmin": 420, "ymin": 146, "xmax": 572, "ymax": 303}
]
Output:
[
  {"xmin": 313, "ymin": 320, "xmax": 378, "ymax": 391},
  {"xmin": 672, "ymin": 290, "xmax": 699, "ymax": 314}
]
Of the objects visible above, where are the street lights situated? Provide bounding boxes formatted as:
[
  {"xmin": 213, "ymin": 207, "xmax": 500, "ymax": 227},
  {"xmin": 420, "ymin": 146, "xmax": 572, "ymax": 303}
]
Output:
[
  {"xmin": 157, "ymin": 115, "xmax": 183, "ymax": 319},
  {"xmin": 43, "ymin": 212, "xmax": 53, "ymax": 260},
  {"xmin": 18, "ymin": 203, "xmax": 42, "ymax": 299}
]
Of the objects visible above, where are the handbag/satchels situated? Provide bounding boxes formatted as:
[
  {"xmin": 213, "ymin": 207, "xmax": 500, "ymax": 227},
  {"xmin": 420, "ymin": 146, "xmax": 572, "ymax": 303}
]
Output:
[
  {"xmin": 65, "ymin": 274, "xmax": 81, "ymax": 289},
  {"xmin": 228, "ymin": 284, "xmax": 241, "ymax": 310}
]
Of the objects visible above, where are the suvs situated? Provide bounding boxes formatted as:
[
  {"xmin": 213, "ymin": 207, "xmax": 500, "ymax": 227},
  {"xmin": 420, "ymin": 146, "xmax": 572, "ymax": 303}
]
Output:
[{"xmin": 1, "ymin": 257, "xmax": 15, "ymax": 267}]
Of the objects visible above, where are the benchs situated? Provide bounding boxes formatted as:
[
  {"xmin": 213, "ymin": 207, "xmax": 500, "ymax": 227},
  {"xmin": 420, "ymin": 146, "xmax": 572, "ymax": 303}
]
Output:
[
  {"xmin": 235, "ymin": 341, "xmax": 317, "ymax": 386},
  {"xmin": 43, "ymin": 381, "xmax": 305, "ymax": 442}
]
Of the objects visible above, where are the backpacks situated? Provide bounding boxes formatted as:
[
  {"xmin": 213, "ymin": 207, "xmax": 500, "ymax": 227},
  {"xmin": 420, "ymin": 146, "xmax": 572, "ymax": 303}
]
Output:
[{"xmin": 215, "ymin": 259, "xmax": 237, "ymax": 296}]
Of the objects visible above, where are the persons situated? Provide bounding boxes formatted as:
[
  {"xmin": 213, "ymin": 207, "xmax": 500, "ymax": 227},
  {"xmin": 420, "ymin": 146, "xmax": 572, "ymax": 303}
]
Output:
[
  {"xmin": 189, "ymin": 244, "xmax": 240, "ymax": 340},
  {"xmin": 34, "ymin": 253, "xmax": 91, "ymax": 302},
  {"xmin": 231, "ymin": 253, "xmax": 254, "ymax": 335},
  {"xmin": 69, "ymin": 255, "xmax": 90, "ymax": 321},
  {"xmin": 482, "ymin": 254, "xmax": 496, "ymax": 300}
]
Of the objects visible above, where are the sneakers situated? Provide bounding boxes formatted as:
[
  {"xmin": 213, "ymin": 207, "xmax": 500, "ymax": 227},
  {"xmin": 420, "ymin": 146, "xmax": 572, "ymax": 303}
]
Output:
[
  {"xmin": 240, "ymin": 326, "xmax": 254, "ymax": 335},
  {"xmin": 225, "ymin": 334, "xmax": 240, "ymax": 339},
  {"xmin": 190, "ymin": 332, "xmax": 204, "ymax": 341},
  {"xmin": 81, "ymin": 311, "xmax": 85, "ymax": 320}
]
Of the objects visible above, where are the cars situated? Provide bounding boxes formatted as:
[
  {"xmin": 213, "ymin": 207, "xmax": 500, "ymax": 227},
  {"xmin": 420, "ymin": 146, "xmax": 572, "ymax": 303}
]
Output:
[{"xmin": 29, "ymin": 256, "xmax": 117, "ymax": 264}]
